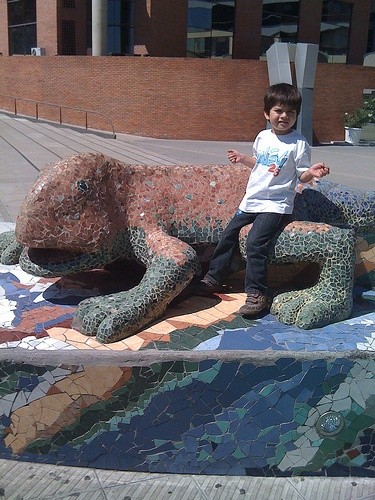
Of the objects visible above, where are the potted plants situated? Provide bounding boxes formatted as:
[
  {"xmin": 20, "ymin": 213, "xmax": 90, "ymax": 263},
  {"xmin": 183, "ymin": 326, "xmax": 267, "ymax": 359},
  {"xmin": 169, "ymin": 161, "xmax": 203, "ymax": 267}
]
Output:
[{"xmin": 342, "ymin": 95, "xmax": 375, "ymax": 145}]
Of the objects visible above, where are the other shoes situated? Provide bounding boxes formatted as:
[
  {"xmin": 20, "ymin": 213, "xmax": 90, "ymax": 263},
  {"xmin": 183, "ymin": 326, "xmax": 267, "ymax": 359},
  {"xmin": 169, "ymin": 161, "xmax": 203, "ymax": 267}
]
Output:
[
  {"xmin": 195, "ymin": 278, "xmax": 229, "ymax": 295},
  {"xmin": 239, "ymin": 292, "xmax": 275, "ymax": 315}
]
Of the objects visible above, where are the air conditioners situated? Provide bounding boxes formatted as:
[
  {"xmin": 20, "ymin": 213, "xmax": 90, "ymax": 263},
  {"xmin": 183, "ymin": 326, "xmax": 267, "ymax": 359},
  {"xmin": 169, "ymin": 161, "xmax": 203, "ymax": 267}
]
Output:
[{"xmin": 31, "ymin": 48, "xmax": 45, "ymax": 56}]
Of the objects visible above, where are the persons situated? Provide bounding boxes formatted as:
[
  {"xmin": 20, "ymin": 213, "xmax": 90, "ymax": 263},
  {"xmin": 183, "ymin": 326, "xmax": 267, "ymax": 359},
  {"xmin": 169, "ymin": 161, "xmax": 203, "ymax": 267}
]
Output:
[{"xmin": 196, "ymin": 82, "xmax": 330, "ymax": 316}]
